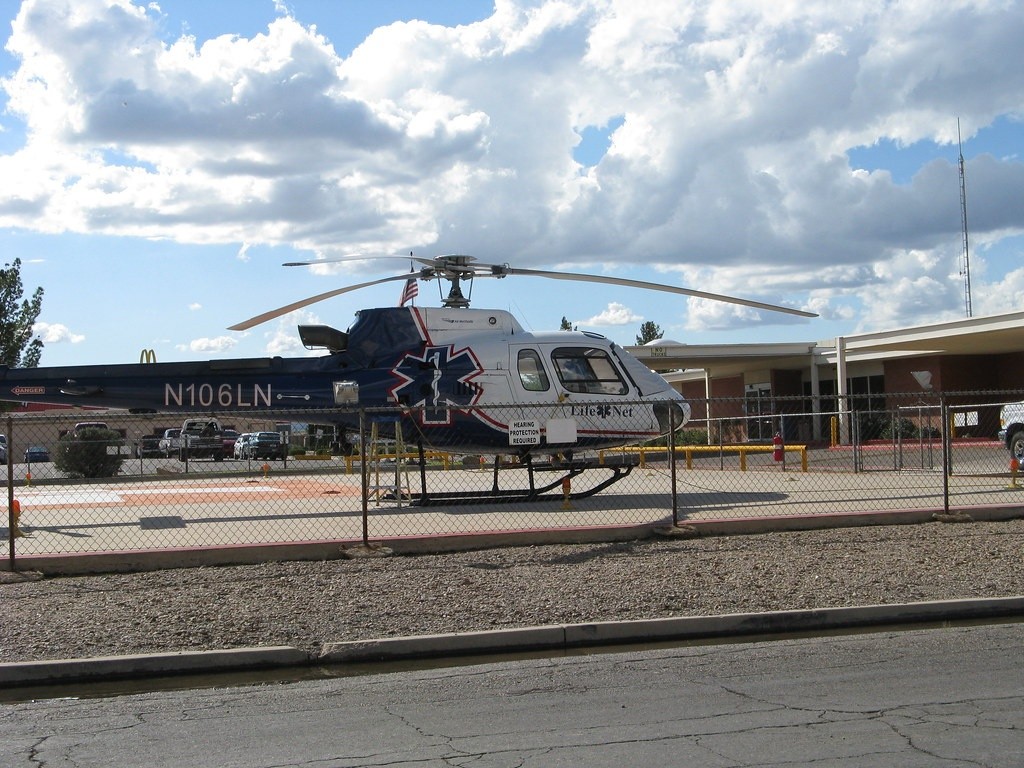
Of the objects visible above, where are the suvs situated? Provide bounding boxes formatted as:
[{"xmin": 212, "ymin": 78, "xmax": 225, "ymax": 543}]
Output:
[{"xmin": 66, "ymin": 422, "xmax": 113, "ymax": 443}]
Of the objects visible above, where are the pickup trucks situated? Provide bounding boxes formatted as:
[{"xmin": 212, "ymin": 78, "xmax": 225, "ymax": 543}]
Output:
[{"xmin": 179, "ymin": 417, "xmax": 225, "ymax": 462}]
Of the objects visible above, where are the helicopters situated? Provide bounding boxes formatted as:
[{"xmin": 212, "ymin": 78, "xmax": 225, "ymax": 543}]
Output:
[{"xmin": 227, "ymin": 252, "xmax": 819, "ymax": 507}]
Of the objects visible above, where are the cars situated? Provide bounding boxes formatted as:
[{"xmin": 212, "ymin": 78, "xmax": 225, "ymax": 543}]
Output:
[
  {"xmin": 998, "ymin": 400, "xmax": 1024, "ymax": 472},
  {"xmin": 159, "ymin": 428, "xmax": 183, "ymax": 459},
  {"xmin": 222, "ymin": 429, "xmax": 253, "ymax": 460},
  {"xmin": 329, "ymin": 432, "xmax": 370, "ymax": 456},
  {"xmin": 23, "ymin": 445, "xmax": 52, "ymax": 463},
  {"xmin": 0, "ymin": 435, "xmax": 9, "ymax": 466},
  {"xmin": 241, "ymin": 431, "xmax": 290, "ymax": 463},
  {"xmin": 134, "ymin": 433, "xmax": 161, "ymax": 459}
]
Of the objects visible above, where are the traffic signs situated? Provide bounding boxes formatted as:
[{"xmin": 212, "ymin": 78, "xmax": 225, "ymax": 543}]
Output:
[{"xmin": 10, "ymin": 385, "xmax": 45, "ymax": 395}]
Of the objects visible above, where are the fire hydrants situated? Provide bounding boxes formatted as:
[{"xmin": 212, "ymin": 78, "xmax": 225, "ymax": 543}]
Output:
[{"xmin": 773, "ymin": 431, "xmax": 785, "ymax": 462}]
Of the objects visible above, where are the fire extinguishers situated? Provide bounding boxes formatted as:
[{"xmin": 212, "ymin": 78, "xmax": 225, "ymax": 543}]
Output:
[{"xmin": 773, "ymin": 432, "xmax": 784, "ymax": 462}]
